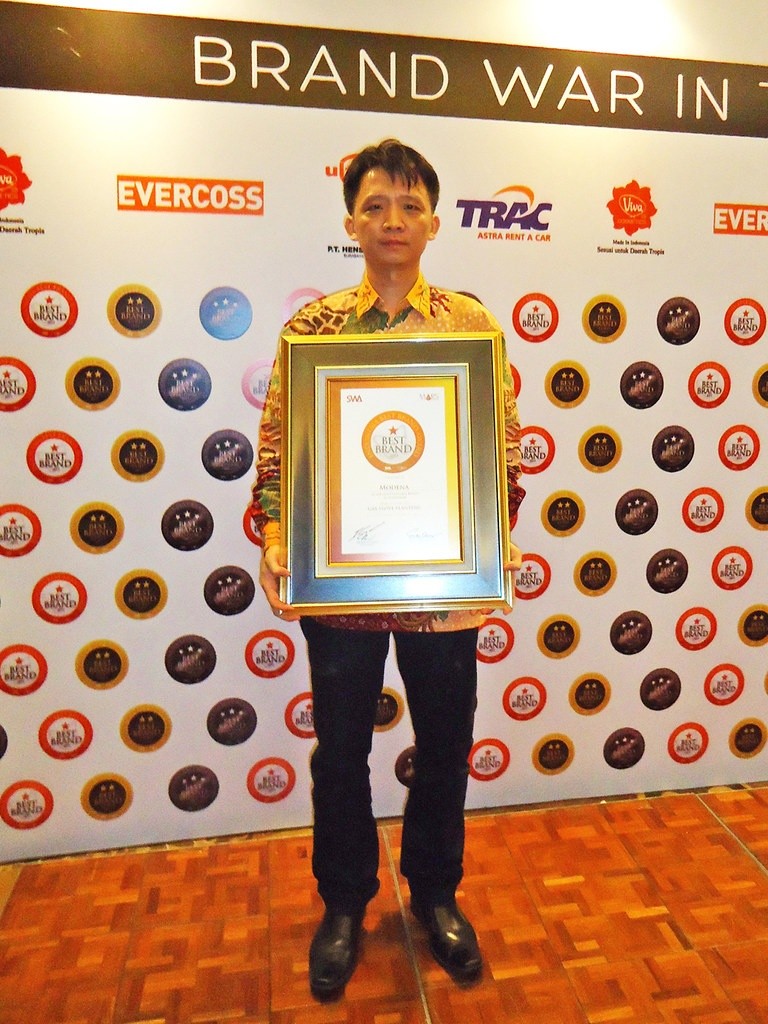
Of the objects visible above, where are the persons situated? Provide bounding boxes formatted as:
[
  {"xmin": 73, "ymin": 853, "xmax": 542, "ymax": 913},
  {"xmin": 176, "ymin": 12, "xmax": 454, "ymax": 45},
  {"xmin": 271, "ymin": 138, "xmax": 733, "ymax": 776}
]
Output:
[{"xmin": 252, "ymin": 140, "xmax": 522, "ymax": 1003}]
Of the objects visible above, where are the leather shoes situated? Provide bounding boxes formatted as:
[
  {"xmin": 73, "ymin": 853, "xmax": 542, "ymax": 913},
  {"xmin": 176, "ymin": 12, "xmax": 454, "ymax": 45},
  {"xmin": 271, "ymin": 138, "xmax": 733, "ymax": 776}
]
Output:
[
  {"xmin": 309, "ymin": 907, "xmax": 363, "ymax": 991},
  {"xmin": 410, "ymin": 892, "xmax": 483, "ymax": 972}
]
